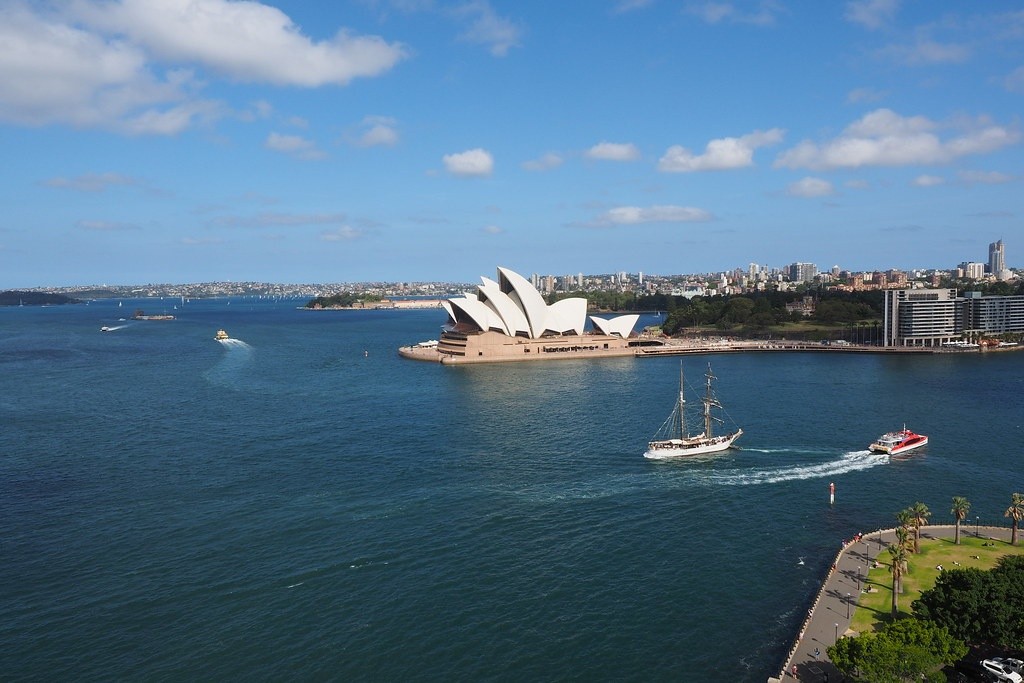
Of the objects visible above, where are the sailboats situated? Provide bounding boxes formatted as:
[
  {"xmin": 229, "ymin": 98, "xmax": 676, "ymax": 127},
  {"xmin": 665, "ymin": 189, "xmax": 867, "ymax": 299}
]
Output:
[{"xmin": 642, "ymin": 359, "xmax": 744, "ymax": 460}]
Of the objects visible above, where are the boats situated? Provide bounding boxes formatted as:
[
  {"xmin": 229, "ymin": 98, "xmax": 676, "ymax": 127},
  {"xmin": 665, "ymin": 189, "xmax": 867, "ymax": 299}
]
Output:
[
  {"xmin": 216, "ymin": 329, "xmax": 229, "ymax": 341},
  {"xmin": 869, "ymin": 422, "xmax": 928, "ymax": 456},
  {"xmin": 101, "ymin": 325, "xmax": 110, "ymax": 332}
]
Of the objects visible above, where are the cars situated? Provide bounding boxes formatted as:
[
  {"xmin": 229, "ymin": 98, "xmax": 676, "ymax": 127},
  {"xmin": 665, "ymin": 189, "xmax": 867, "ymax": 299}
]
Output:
[{"xmin": 982, "ymin": 657, "xmax": 1024, "ymax": 683}]
